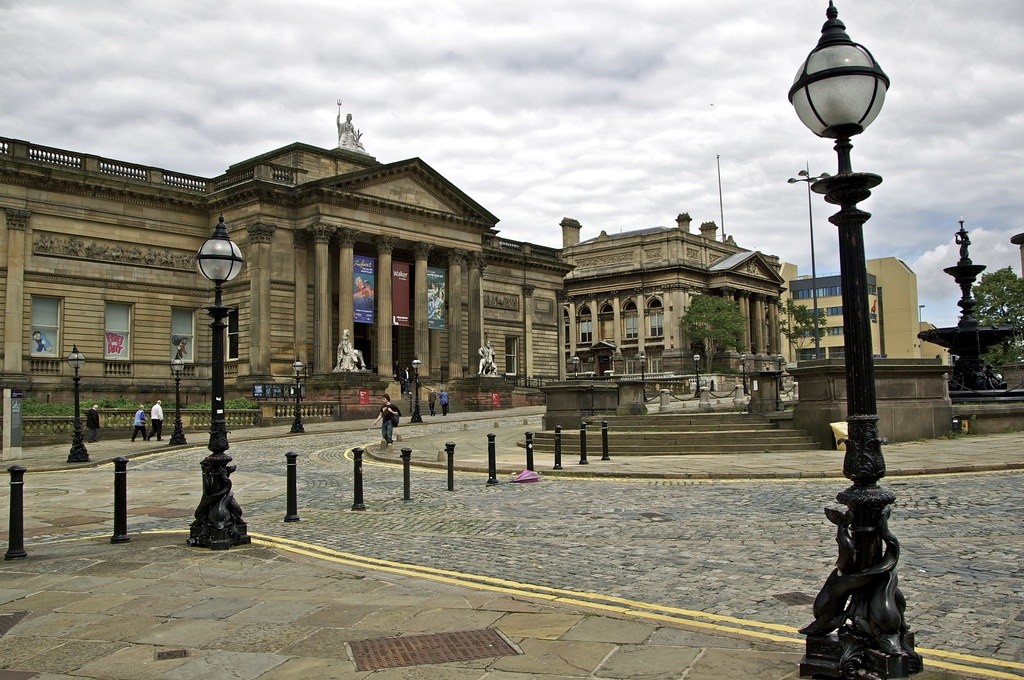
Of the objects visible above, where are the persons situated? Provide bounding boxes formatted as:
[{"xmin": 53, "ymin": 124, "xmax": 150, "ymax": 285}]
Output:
[
  {"xmin": 401, "ymin": 367, "xmax": 413, "ymax": 394},
  {"xmin": 131, "ymin": 404, "xmax": 147, "ymax": 441},
  {"xmin": 337, "ymin": 329, "xmax": 368, "ymax": 372},
  {"xmin": 478, "ymin": 340, "xmax": 498, "ymax": 376},
  {"xmin": 374, "ymin": 394, "xmax": 399, "ymax": 445},
  {"xmin": 427, "ymin": 280, "xmax": 444, "ymax": 321},
  {"xmin": 438, "ymin": 389, "xmax": 450, "ymax": 416},
  {"xmin": 86, "ymin": 403, "xmax": 100, "ymax": 444},
  {"xmin": 337, "ymin": 114, "xmax": 365, "ymax": 152},
  {"xmin": 428, "ymin": 388, "xmax": 437, "ymax": 416},
  {"xmin": 148, "ymin": 400, "xmax": 163, "ymax": 442},
  {"xmin": 394, "ymin": 360, "xmax": 401, "ymax": 382}
]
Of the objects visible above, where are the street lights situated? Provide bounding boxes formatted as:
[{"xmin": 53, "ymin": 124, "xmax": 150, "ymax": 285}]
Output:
[
  {"xmin": 783, "ymin": 1, "xmax": 925, "ymax": 680},
  {"xmin": 571, "ymin": 354, "xmax": 580, "ymax": 376},
  {"xmin": 64, "ymin": 342, "xmax": 91, "ymax": 463},
  {"xmin": 692, "ymin": 352, "xmax": 702, "ymax": 399},
  {"xmin": 638, "ymin": 353, "xmax": 648, "ymax": 402},
  {"xmin": 185, "ymin": 211, "xmax": 251, "ymax": 550},
  {"xmin": 785, "ymin": 158, "xmax": 831, "ymax": 360},
  {"xmin": 410, "ymin": 353, "xmax": 425, "ymax": 423},
  {"xmin": 170, "ymin": 353, "xmax": 188, "ymax": 444},
  {"xmin": 288, "ymin": 355, "xmax": 307, "ymax": 433},
  {"xmin": 918, "ymin": 304, "xmax": 925, "ymax": 334},
  {"xmin": 737, "ymin": 353, "xmax": 751, "ymax": 396}
]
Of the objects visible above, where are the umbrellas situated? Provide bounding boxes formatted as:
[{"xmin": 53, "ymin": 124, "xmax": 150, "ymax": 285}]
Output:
[{"xmin": 513, "ymin": 470, "xmax": 539, "ymax": 482}]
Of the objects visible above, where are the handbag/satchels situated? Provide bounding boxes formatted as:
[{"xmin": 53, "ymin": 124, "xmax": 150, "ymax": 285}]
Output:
[
  {"xmin": 390, "ymin": 410, "xmax": 399, "ymax": 428},
  {"xmin": 141, "ymin": 418, "xmax": 146, "ymax": 424}
]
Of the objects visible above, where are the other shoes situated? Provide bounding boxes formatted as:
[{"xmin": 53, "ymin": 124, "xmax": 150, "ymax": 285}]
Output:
[
  {"xmin": 148, "ymin": 435, "xmax": 150, "ymax": 441},
  {"xmin": 131, "ymin": 439, "xmax": 135, "ymax": 442},
  {"xmin": 434, "ymin": 412, "xmax": 436, "ymax": 416},
  {"xmin": 89, "ymin": 439, "xmax": 98, "ymax": 443},
  {"xmin": 157, "ymin": 438, "xmax": 163, "ymax": 441}
]
